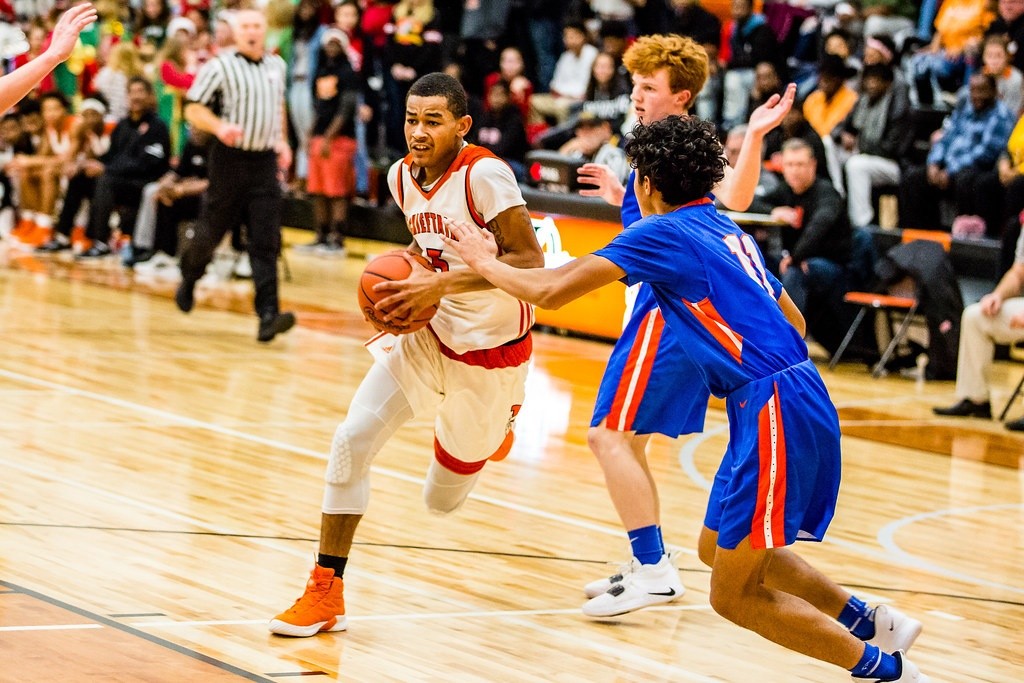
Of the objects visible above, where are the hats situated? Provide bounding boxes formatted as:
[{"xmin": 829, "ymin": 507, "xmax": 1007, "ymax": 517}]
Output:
[
  {"xmin": 817, "ymin": 53, "xmax": 858, "ymax": 80},
  {"xmin": 321, "ymin": 25, "xmax": 351, "ymax": 47}
]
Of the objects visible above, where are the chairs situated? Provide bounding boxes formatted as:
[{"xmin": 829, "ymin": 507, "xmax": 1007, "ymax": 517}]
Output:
[{"xmin": 826, "ymin": 230, "xmax": 953, "ymax": 376}]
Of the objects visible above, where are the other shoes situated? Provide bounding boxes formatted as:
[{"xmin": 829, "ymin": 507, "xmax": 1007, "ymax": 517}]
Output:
[
  {"xmin": 234, "ymin": 252, "xmax": 252, "ymax": 278},
  {"xmin": 294, "ymin": 242, "xmax": 347, "ymax": 260}
]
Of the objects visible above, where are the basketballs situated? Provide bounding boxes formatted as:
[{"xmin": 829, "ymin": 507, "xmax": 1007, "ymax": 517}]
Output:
[{"xmin": 358, "ymin": 249, "xmax": 441, "ymax": 335}]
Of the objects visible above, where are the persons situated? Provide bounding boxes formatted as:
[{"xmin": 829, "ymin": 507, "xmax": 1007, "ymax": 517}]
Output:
[
  {"xmin": 437, "ymin": 114, "xmax": 938, "ymax": 683},
  {"xmin": 0, "ymin": 2, "xmax": 98, "ymax": 118},
  {"xmin": 174, "ymin": 10, "xmax": 294, "ymax": 343},
  {"xmin": 577, "ymin": 31, "xmax": 797, "ymax": 617},
  {"xmin": 0, "ymin": 0, "xmax": 1024, "ymax": 364},
  {"xmin": 269, "ymin": 72, "xmax": 545, "ymax": 637},
  {"xmin": 932, "ymin": 223, "xmax": 1024, "ymax": 431}
]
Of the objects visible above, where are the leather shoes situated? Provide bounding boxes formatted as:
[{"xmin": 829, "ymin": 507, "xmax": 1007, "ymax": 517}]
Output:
[
  {"xmin": 933, "ymin": 398, "xmax": 991, "ymax": 418},
  {"xmin": 1004, "ymin": 418, "xmax": 1024, "ymax": 432}
]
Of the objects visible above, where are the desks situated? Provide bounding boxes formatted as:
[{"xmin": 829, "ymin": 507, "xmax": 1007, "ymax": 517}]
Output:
[{"xmin": 521, "ymin": 186, "xmax": 790, "ymax": 346}]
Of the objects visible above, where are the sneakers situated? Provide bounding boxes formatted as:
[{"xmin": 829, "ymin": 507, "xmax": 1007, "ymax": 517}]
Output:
[
  {"xmin": 4, "ymin": 217, "xmax": 56, "ymax": 252},
  {"xmin": 582, "ymin": 550, "xmax": 685, "ymax": 617},
  {"xmin": 267, "ymin": 562, "xmax": 348, "ymax": 637},
  {"xmin": 851, "ymin": 650, "xmax": 930, "ymax": 683},
  {"xmin": 176, "ymin": 280, "xmax": 195, "ymax": 312},
  {"xmin": 122, "ymin": 249, "xmax": 181, "ymax": 275},
  {"xmin": 862, "ymin": 603, "xmax": 922, "ymax": 655},
  {"xmin": 257, "ymin": 313, "xmax": 295, "ymax": 342},
  {"xmin": 488, "ymin": 390, "xmax": 525, "ymax": 463},
  {"xmin": 34, "ymin": 238, "xmax": 74, "ymax": 255},
  {"xmin": 74, "ymin": 239, "xmax": 114, "ymax": 262}
]
